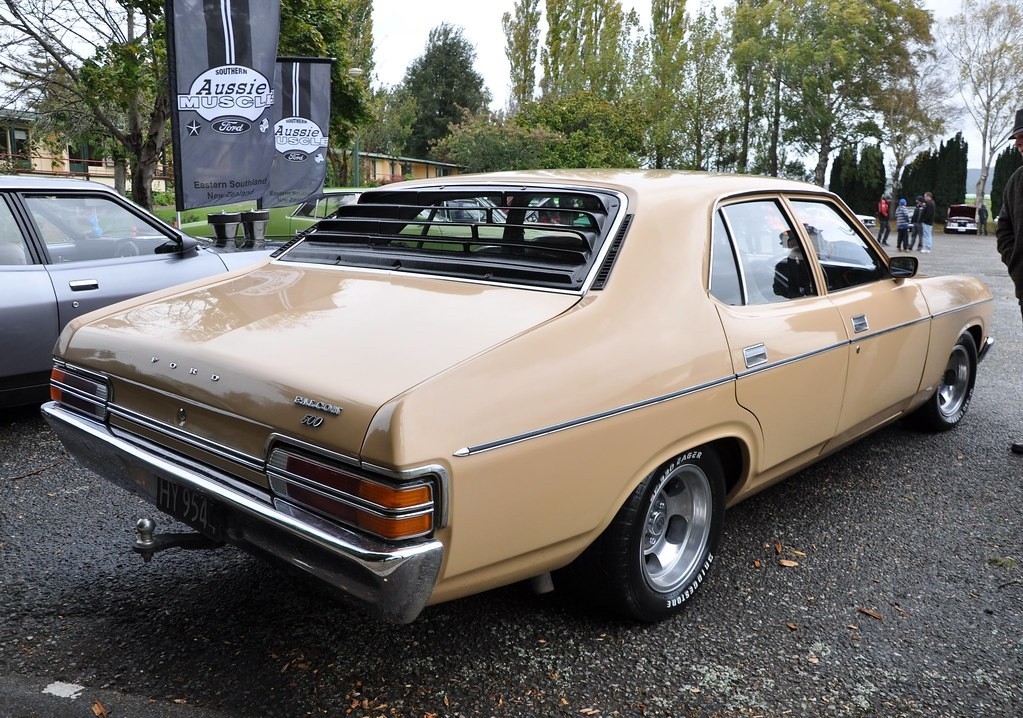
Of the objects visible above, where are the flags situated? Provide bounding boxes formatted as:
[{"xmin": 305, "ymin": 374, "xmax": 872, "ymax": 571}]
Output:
[{"xmin": 168, "ymin": 0, "xmax": 330, "ymax": 211}]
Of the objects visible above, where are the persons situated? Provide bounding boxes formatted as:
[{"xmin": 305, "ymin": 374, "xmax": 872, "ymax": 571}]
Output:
[
  {"xmin": 171, "ymin": 217, "xmax": 178, "ymax": 227},
  {"xmin": 990, "ymin": 109, "xmax": 1023, "ymax": 451},
  {"xmin": 906, "ymin": 196, "xmax": 924, "ymax": 252},
  {"xmin": 978, "ymin": 204, "xmax": 988, "ymax": 235},
  {"xmin": 894, "ymin": 199, "xmax": 909, "ymax": 253},
  {"xmin": 878, "ymin": 193, "xmax": 892, "ymax": 247},
  {"xmin": 921, "ymin": 192, "xmax": 934, "ymax": 253}
]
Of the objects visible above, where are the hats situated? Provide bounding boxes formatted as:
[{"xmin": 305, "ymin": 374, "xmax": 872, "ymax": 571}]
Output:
[
  {"xmin": 917, "ymin": 196, "xmax": 923, "ymax": 201},
  {"xmin": 899, "ymin": 199, "xmax": 906, "ymax": 205},
  {"xmin": 883, "ymin": 193, "xmax": 892, "ymax": 199},
  {"xmin": 1008, "ymin": 108, "xmax": 1023, "ymax": 139}
]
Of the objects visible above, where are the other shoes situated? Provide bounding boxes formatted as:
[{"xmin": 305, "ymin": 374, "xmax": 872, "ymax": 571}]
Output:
[
  {"xmin": 897, "ymin": 248, "xmax": 903, "ymax": 253},
  {"xmin": 1011, "ymin": 442, "xmax": 1023, "ymax": 455},
  {"xmin": 921, "ymin": 249, "xmax": 930, "ymax": 253},
  {"xmin": 882, "ymin": 242, "xmax": 889, "ymax": 246},
  {"xmin": 908, "ymin": 246, "xmax": 912, "ymax": 250},
  {"xmin": 917, "ymin": 247, "xmax": 922, "ymax": 251},
  {"xmin": 903, "ymin": 249, "xmax": 910, "ymax": 252}
]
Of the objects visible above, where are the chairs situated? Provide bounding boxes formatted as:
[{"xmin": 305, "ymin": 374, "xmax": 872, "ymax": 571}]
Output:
[{"xmin": 522, "ymin": 235, "xmax": 587, "ymax": 265}]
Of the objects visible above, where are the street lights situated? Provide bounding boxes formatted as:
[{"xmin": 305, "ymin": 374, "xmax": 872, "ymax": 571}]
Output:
[{"xmin": 347, "ymin": 68, "xmax": 364, "ymax": 189}]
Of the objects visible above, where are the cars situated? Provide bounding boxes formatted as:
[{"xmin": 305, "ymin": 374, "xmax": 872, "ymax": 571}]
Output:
[
  {"xmin": 38, "ymin": 167, "xmax": 997, "ymax": 624},
  {"xmin": 173, "ymin": 185, "xmax": 593, "ymax": 254},
  {"xmin": 943, "ymin": 203, "xmax": 980, "ymax": 235},
  {"xmin": 905, "ymin": 206, "xmax": 917, "ymax": 229},
  {"xmin": 0, "ymin": 173, "xmax": 288, "ymax": 398},
  {"xmin": 855, "ymin": 214, "xmax": 879, "ymax": 228}
]
